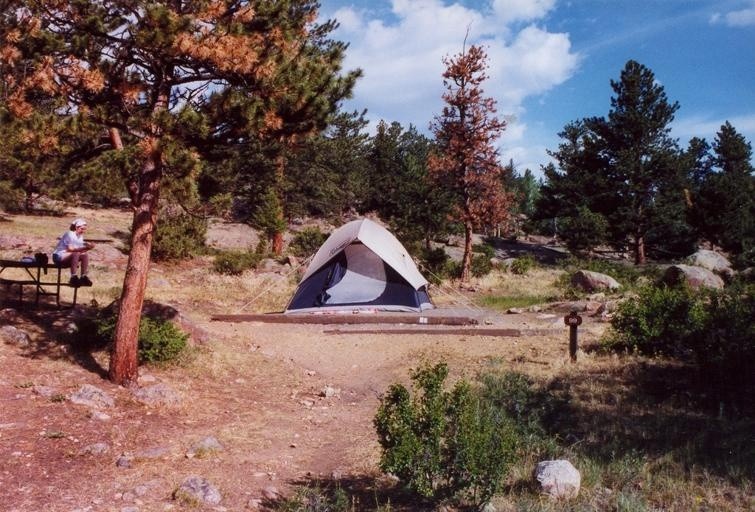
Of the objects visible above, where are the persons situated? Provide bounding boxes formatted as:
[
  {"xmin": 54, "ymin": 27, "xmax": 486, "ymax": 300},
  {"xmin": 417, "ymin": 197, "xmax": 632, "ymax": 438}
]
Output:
[{"xmin": 51, "ymin": 218, "xmax": 94, "ymax": 287}]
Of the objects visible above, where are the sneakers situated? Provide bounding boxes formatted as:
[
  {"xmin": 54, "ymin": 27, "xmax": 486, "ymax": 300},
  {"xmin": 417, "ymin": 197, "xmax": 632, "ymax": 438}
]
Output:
[
  {"xmin": 70, "ymin": 276, "xmax": 80, "ymax": 287},
  {"xmin": 80, "ymin": 276, "xmax": 92, "ymax": 286}
]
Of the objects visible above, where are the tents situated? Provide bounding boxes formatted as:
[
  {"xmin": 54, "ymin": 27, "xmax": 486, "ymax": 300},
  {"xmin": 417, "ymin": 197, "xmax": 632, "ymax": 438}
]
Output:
[{"xmin": 283, "ymin": 218, "xmax": 435, "ymax": 315}]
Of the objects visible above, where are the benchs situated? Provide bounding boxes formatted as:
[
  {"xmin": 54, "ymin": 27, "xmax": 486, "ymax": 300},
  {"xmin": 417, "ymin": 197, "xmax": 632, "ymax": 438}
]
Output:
[{"xmin": 0, "ymin": 277, "xmax": 77, "ymax": 308}]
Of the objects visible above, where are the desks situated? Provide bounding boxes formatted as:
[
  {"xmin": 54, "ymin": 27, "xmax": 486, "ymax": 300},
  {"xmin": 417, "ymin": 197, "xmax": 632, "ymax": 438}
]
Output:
[{"xmin": 0, "ymin": 260, "xmax": 78, "ymax": 309}]
